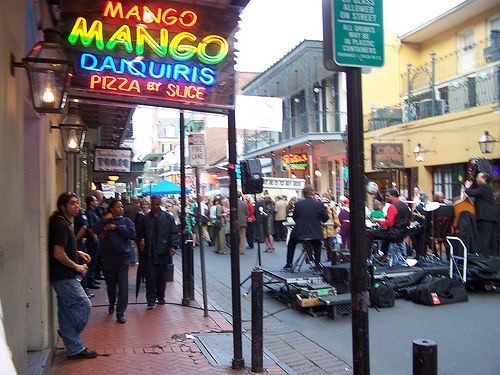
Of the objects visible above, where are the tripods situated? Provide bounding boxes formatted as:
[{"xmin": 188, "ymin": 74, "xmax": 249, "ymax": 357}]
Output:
[{"xmin": 241, "ymin": 193, "xmax": 280, "ymax": 295}]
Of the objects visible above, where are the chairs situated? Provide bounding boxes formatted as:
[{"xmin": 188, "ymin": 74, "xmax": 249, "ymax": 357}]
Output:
[{"xmin": 427, "ymin": 215, "xmax": 450, "ymax": 260}]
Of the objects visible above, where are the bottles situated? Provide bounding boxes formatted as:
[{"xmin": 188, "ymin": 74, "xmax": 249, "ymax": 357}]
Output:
[{"xmin": 76, "ymin": 263, "xmax": 87, "ymax": 281}]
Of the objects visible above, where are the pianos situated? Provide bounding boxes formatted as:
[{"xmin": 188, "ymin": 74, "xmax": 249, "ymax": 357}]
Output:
[{"xmin": 281, "ymin": 222, "xmax": 336, "ymax": 272}]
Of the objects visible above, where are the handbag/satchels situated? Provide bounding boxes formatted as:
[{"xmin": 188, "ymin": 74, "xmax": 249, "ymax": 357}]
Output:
[
  {"xmin": 102, "ymin": 231, "xmax": 130, "ymax": 255},
  {"xmin": 214, "ymin": 221, "xmax": 221, "ymax": 229},
  {"xmin": 247, "ymin": 217, "xmax": 252, "ymax": 222},
  {"xmin": 370, "ymin": 282, "xmax": 394, "ymax": 308},
  {"xmin": 412, "ymin": 276, "xmax": 469, "ymax": 305}
]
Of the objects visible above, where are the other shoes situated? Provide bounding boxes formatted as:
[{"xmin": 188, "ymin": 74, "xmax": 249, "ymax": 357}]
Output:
[
  {"xmin": 158, "ymin": 297, "xmax": 165, "ymax": 304},
  {"xmin": 109, "ymin": 304, "xmax": 114, "ymax": 314},
  {"xmin": 316, "ymin": 265, "xmax": 322, "ymax": 269},
  {"xmin": 147, "ymin": 301, "xmax": 156, "ymax": 308},
  {"xmin": 97, "ymin": 275, "xmax": 105, "ymax": 279},
  {"xmin": 68, "ymin": 348, "xmax": 97, "ymax": 358},
  {"xmin": 95, "ymin": 280, "xmax": 101, "ymax": 284},
  {"xmin": 57, "ymin": 329, "xmax": 63, "ymax": 338},
  {"xmin": 117, "ymin": 315, "xmax": 125, "ymax": 323},
  {"xmin": 87, "ymin": 284, "xmax": 100, "ymax": 288},
  {"xmin": 284, "ymin": 264, "xmax": 292, "ymax": 270}
]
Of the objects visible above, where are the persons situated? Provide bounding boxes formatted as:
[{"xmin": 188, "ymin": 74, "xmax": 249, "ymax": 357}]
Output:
[
  {"xmin": 464, "ymin": 171, "xmax": 498, "ymax": 257},
  {"xmin": 314, "ymin": 188, "xmax": 351, "ymax": 266},
  {"xmin": 48, "ymin": 192, "xmax": 99, "ymax": 358},
  {"xmin": 283, "ymin": 185, "xmax": 330, "ymax": 271},
  {"xmin": 364, "ymin": 184, "xmax": 456, "ymax": 262},
  {"xmin": 74, "ymin": 189, "xmax": 180, "ymax": 324},
  {"xmin": 236, "ymin": 191, "xmax": 296, "ymax": 254},
  {"xmin": 159, "ymin": 195, "xmax": 231, "ymax": 255}
]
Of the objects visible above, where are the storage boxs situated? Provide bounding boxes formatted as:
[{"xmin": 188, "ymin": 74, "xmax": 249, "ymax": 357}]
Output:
[{"xmin": 297, "ymin": 293, "xmax": 321, "ymax": 307}]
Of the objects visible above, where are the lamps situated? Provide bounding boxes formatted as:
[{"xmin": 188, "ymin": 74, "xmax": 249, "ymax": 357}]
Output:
[
  {"xmin": 50, "ymin": 101, "xmax": 90, "ymax": 154},
  {"xmin": 414, "ymin": 143, "xmax": 438, "ymax": 162},
  {"xmin": 10, "ymin": 27, "xmax": 75, "ymax": 114},
  {"xmin": 477, "ymin": 131, "xmax": 500, "ymax": 154}
]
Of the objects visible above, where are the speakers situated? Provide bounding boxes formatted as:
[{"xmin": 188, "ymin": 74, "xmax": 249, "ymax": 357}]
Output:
[{"xmin": 241, "ymin": 159, "xmax": 264, "ymax": 194}]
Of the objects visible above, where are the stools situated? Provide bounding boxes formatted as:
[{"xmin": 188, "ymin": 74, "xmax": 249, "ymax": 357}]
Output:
[
  {"xmin": 292, "ymin": 235, "xmax": 325, "ymax": 277},
  {"xmin": 385, "ymin": 234, "xmax": 413, "ymax": 269}
]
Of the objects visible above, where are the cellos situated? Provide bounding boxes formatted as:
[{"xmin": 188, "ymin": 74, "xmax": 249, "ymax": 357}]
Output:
[{"xmin": 450, "ymin": 159, "xmax": 477, "ymax": 234}]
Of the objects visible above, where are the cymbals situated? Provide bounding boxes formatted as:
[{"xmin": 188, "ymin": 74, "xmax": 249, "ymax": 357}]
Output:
[{"xmin": 422, "ymin": 203, "xmax": 440, "ymax": 211}]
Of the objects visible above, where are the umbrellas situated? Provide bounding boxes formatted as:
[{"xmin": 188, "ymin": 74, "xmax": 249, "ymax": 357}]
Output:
[{"xmin": 142, "ymin": 179, "xmax": 192, "ymax": 198}]
